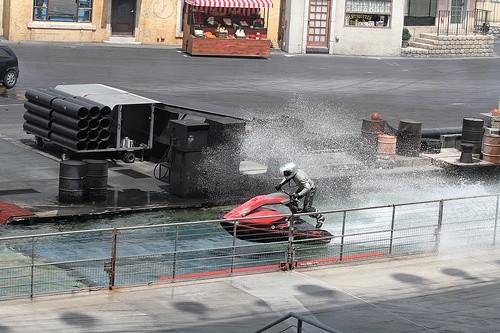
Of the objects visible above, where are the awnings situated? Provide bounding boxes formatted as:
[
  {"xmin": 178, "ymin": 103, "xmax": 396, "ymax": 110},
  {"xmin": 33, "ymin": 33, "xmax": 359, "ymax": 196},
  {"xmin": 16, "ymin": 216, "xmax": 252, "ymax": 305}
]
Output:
[{"xmin": 184, "ymin": 0, "xmax": 274, "ymax": 15}]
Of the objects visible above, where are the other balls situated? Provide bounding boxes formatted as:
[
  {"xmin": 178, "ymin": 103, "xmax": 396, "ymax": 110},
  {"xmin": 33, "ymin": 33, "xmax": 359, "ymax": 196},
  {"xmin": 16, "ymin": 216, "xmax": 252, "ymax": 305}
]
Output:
[
  {"xmin": 492, "ymin": 108, "xmax": 499, "ymax": 115},
  {"xmin": 371, "ymin": 112, "xmax": 380, "ymax": 120}
]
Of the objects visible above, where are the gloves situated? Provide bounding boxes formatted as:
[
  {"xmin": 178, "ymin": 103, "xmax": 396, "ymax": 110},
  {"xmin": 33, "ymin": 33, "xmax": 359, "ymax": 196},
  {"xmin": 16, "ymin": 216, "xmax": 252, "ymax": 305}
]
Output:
[
  {"xmin": 276, "ymin": 184, "xmax": 282, "ymax": 191},
  {"xmin": 293, "ymin": 194, "xmax": 300, "ymax": 200}
]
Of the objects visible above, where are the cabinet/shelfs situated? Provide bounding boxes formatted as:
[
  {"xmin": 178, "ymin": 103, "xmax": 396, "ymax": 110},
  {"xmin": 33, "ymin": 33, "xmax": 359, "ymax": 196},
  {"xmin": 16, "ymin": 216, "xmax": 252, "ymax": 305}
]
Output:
[{"xmin": 182, "ymin": 12, "xmax": 271, "ymax": 57}]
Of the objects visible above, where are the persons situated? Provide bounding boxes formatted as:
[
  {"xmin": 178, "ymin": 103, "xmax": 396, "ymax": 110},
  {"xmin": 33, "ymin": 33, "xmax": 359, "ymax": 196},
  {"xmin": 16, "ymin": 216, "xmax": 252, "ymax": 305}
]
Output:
[{"xmin": 275, "ymin": 162, "xmax": 325, "ymax": 228}]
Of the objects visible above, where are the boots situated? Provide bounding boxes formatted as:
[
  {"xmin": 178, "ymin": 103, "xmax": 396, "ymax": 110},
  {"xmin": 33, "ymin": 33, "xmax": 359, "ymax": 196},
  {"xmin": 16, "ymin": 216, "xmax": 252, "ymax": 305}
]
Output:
[{"xmin": 311, "ymin": 209, "xmax": 325, "ymax": 228}]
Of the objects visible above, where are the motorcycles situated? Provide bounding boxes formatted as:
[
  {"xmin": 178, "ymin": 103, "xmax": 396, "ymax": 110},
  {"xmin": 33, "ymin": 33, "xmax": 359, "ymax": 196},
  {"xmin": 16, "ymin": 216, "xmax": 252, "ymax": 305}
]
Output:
[{"xmin": 220, "ymin": 188, "xmax": 336, "ymax": 245}]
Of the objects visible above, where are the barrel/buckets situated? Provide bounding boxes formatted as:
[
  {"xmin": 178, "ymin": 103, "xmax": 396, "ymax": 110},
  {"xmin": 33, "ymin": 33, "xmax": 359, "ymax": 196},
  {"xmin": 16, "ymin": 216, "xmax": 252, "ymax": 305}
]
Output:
[
  {"xmin": 83, "ymin": 159, "xmax": 108, "ymax": 202},
  {"xmin": 397, "ymin": 120, "xmax": 422, "ymax": 156},
  {"xmin": 482, "ymin": 134, "xmax": 500, "ymax": 164},
  {"xmin": 461, "ymin": 117, "xmax": 484, "ymax": 155},
  {"xmin": 361, "ymin": 119, "xmax": 385, "ymax": 141},
  {"xmin": 59, "ymin": 160, "xmax": 87, "ymax": 203}
]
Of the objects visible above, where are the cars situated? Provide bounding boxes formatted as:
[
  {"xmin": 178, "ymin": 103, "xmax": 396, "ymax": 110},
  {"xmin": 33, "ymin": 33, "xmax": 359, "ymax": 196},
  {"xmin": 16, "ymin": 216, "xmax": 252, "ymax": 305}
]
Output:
[{"xmin": 0, "ymin": 45, "xmax": 20, "ymax": 89}]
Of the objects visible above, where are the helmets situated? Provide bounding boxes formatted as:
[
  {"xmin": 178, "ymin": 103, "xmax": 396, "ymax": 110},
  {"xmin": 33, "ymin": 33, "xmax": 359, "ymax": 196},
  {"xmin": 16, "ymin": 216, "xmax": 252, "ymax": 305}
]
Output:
[{"xmin": 283, "ymin": 163, "xmax": 298, "ymax": 179}]
